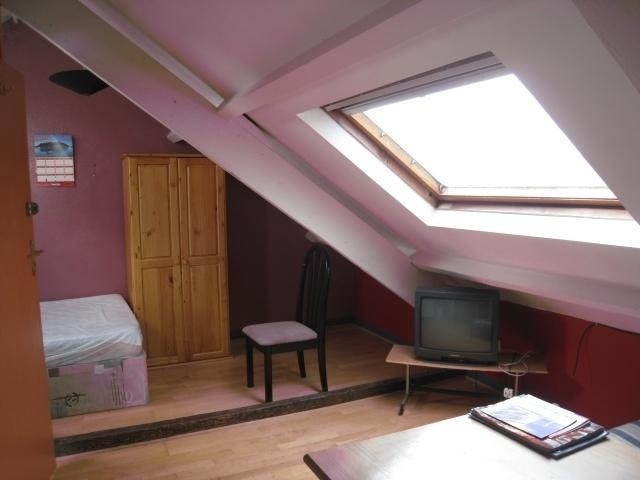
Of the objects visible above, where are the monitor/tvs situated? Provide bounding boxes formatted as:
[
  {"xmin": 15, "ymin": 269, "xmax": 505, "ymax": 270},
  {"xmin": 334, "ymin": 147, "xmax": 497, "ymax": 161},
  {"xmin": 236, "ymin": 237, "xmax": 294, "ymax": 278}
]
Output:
[{"xmin": 414, "ymin": 287, "xmax": 501, "ymax": 365}]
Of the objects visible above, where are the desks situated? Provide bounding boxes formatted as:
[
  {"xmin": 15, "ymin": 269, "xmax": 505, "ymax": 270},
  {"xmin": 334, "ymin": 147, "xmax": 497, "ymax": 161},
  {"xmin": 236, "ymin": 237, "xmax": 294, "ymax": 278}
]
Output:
[
  {"xmin": 385, "ymin": 342, "xmax": 550, "ymax": 417},
  {"xmin": 303, "ymin": 403, "xmax": 639, "ymax": 480}
]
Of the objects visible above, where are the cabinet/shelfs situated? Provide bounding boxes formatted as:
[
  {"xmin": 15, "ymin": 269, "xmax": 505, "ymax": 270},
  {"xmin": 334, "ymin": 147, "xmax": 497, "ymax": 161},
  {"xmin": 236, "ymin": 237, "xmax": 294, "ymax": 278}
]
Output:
[{"xmin": 118, "ymin": 152, "xmax": 234, "ymax": 369}]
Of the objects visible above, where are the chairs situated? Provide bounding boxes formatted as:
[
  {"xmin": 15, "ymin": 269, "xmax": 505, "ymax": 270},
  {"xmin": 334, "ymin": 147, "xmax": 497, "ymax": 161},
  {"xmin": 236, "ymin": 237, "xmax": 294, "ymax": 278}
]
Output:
[{"xmin": 239, "ymin": 244, "xmax": 331, "ymax": 403}]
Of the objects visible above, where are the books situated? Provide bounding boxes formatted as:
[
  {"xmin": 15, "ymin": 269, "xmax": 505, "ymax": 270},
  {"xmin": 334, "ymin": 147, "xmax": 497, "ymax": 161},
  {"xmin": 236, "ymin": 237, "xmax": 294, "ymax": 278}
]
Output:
[{"xmin": 467, "ymin": 395, "xmax": 611, "ymax": 459}]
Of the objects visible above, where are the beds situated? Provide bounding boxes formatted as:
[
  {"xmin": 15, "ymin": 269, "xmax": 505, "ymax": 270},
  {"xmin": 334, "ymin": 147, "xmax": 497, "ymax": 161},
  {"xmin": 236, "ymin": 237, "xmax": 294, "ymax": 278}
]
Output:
[{"xmin": 37, "ymin": 290, "xmax": 150, "ymax": 420}]
